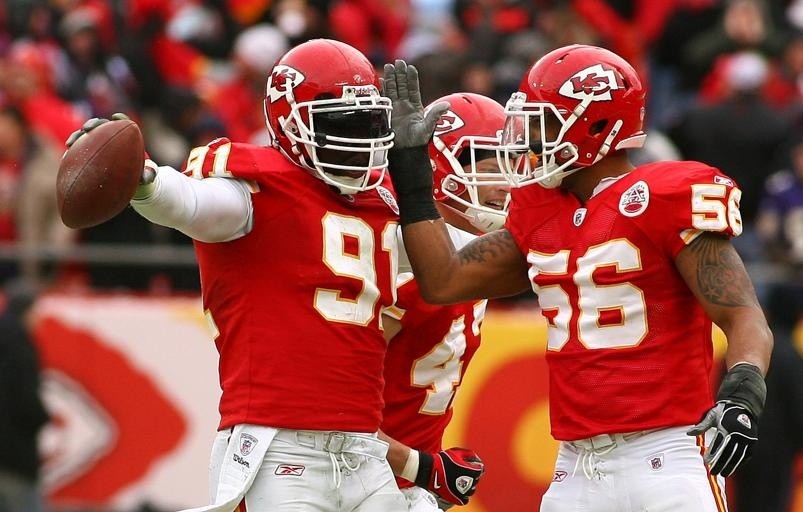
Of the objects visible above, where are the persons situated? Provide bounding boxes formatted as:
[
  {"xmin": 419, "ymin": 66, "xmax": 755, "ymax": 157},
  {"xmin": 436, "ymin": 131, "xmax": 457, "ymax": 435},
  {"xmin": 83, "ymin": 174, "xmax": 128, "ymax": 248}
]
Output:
[
  {"xmin": 55, "ymin": 38, "xmax": 411, "ymax": 512},
  {"xmin": 712, "ymin": 260, "xmax": 803, "ymax": 512},
  {"xmin": 372, "ymin": 1, "xmax": 803, "ymax": 326},
  {"xmin": 380, "ymin": 45, "xmax": 776, "ymax": 512},
  {"xmin": 0, "ymin": 0, "xmax": 414, "ymax": 294},
  {"xmin": 384, "ymin": 94, "xmax": 507, "ymax": 512},
  {"xmin": 0, "ymin": 258, "xmax": 53, "ymax": 512}
]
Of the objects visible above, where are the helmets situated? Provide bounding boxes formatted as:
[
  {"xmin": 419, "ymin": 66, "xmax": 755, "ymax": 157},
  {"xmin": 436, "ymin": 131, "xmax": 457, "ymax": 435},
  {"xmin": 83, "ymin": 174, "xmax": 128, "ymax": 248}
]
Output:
[
  {"xmin": 493, "ymin": 44, "xmax": 646, "ymax": 188},
  {"xmin": 424, "ymin": 91, "xmax": 524, "ymax": 233},
  {"xmin": 263, "ymin": 38, "xmax": 395, "ymax": 194}
]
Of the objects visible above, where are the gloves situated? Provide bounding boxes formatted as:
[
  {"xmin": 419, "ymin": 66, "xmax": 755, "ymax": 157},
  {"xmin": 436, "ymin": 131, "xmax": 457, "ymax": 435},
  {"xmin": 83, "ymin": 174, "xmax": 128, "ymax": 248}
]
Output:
[
  {"xmin": 62, "ymin": 113, "xmax": 158, "ymax": 201},
  {"xmin": 379, "ymin": 59, "xmax": 451, "ymax": 149},
  {"xmin": 686, "ymin": 402, "xmax": 760, "ymax": 477},
  {"xmin": 412, "ymin": 447, "xmax": 484, "ymax": 505}
]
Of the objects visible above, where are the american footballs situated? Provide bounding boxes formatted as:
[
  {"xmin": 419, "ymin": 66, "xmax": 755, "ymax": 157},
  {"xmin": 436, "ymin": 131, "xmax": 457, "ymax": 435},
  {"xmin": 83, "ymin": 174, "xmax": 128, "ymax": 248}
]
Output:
[{"xmin": 56, "ymin": 120, "xmax": 144, "ymax": 228}]
